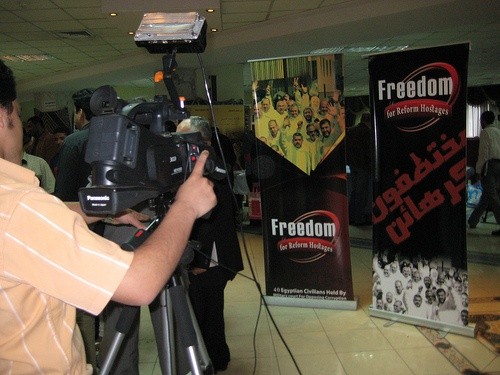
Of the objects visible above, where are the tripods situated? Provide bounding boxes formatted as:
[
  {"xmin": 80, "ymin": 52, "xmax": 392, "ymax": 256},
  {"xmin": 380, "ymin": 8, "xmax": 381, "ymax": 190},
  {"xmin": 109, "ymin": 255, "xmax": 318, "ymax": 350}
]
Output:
[{"xmin": 98, "ymin": 200, "xmax": 214, "ymax": 375}]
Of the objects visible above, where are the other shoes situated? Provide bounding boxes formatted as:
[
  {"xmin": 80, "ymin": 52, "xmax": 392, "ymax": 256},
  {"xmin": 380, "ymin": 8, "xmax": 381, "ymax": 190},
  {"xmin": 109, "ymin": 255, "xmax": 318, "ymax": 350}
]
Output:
[
  {"xmin": 492, "ymin": 228, "xmax": 500, "ymax": 236},
  {"xmin": 466, "ymin": 223, "xmax": 477, "ymax": 230}
]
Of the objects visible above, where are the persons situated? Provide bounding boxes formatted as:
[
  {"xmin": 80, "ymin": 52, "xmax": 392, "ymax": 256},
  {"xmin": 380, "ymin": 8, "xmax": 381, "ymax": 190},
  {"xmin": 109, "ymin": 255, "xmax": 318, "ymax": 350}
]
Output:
[
  {"xmin": 252, "ymin": 76, "xmax": 344, "ymax": 175},
  {"xmin": 1, "ymin": 59, "xmax": 218, "ymax": 374},
  {"xmin": 371, "ymin": 251, "xmax": 469, "ymax": 328},
  {"xmin": 22, "ymin": 87, "xmax": 245, "ymax": 375},
  {"xmin": 466, "ymin": 110, "xmax": 500, "ymax": 237}
]
